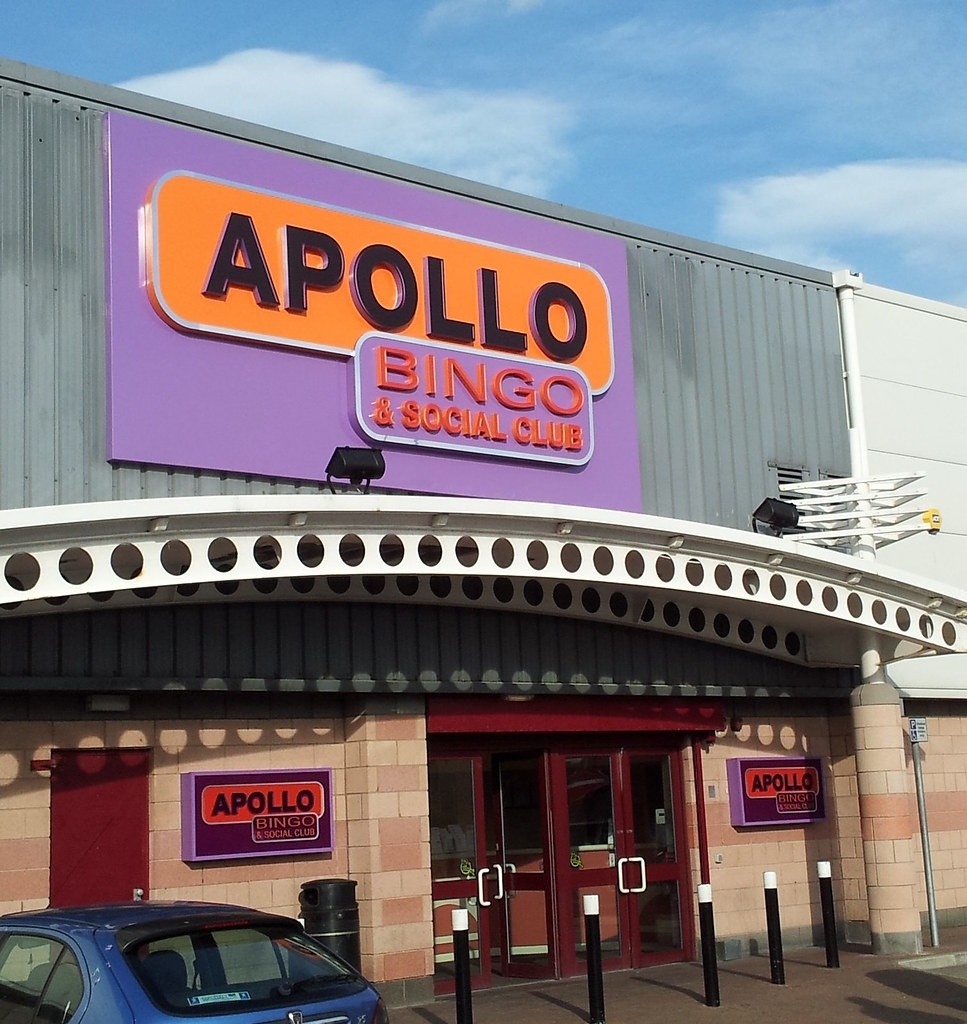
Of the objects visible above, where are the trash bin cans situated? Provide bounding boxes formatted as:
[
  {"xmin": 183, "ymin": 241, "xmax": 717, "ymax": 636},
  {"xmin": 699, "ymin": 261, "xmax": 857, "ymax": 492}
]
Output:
[{"xmin": 298, "ymin": 878, "xmax": 362, "ymax": 975}]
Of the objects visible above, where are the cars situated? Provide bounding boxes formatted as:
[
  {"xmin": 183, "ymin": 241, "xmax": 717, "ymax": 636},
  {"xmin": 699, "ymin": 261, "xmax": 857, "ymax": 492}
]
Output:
[{"xmin": 0, "ymin": 900, "xmax": 388, "ymax": 1024}]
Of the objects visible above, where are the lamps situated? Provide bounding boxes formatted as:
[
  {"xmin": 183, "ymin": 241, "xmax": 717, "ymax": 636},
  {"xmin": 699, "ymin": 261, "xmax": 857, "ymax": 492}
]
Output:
[
  {"xmin": 323, "ymin": 446, "xmax": 387, "ymax": 495},
  {"xmin": 751, "ymin": 498, "xmax": 801, "ymax": 536}
]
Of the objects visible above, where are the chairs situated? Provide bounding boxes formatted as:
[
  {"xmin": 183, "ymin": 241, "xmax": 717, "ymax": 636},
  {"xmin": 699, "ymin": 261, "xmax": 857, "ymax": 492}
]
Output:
[{"xmin": 28, "ymin": 949, "xmax": 193, "ymax": 1011}]
